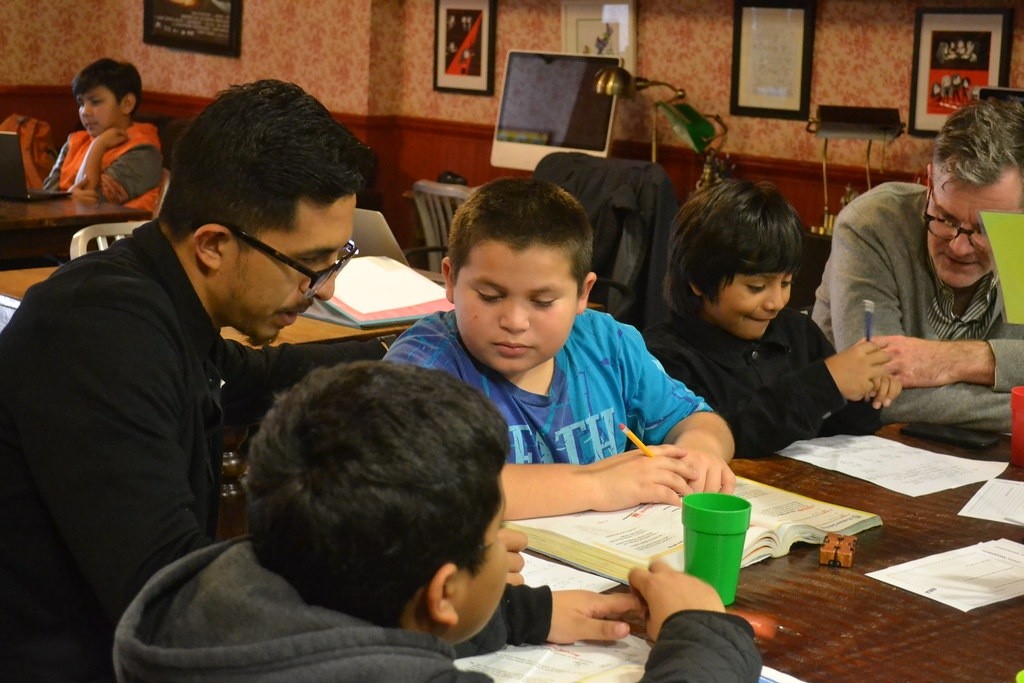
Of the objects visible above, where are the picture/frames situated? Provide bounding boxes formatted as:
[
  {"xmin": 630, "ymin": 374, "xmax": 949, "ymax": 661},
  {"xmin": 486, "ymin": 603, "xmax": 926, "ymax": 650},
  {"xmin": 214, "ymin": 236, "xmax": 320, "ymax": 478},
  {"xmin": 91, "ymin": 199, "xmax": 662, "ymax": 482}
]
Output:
[
  {"xmin": 907, "ymin": 6, "xmax": 1014, "ymax": 138},
  {"xmin": 434, "ymin": 0, "xmax": 496, "ymax": 94},
  {"xmin": 730, "ymin": 1, "xmax": 816, "ymax": 121},
  {"xmin": 559, "ymin": 1, "xmax": 639, "ymax": 79},
  {"xmin": 142, "ymin": 0, "xmax": 242, "ymax": 59}
]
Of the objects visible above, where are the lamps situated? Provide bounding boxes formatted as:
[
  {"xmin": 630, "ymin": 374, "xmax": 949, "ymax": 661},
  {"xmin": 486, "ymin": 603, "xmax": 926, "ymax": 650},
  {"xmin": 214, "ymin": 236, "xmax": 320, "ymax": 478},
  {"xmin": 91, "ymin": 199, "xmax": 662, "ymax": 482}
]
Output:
[
  {"xmin": 661, "ymin": 100, "xmax": 729, "ymax": 193},
  {"xmin": 594, "ymin": 68, "xmax": 685, "ymax": 163},
  {"xmin": 806, "ymin": 120, "xmax": 902, "ymax": 235}
]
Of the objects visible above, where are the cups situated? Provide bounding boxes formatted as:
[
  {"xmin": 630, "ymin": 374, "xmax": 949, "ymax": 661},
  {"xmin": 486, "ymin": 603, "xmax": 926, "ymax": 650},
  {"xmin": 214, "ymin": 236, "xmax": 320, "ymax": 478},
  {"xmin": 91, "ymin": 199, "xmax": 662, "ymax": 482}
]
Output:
[
  {"xmin": 682, "ymin": 493, "xmax": 752, "ymax": 606},
  {"xmin": 1011, "ymin": 386, "xmax": 1024, "ymax": 466}
]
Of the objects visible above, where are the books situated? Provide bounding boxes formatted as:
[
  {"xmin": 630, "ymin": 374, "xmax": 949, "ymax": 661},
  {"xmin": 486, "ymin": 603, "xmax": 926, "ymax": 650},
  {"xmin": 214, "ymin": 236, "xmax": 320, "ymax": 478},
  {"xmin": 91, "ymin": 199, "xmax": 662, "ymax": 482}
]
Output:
[
  {"xmin": 297, "ymin": 255, "xmax": 455, "ymax": 329},
  {"xmin": 503, "ymin": 475, "xmax": 883, "ymax": 589}
]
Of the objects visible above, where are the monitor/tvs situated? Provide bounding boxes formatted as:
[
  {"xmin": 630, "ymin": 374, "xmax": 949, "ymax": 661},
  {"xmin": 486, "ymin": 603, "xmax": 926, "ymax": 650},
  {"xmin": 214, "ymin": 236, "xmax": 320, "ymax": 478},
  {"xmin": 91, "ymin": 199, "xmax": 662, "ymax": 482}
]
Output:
[{"xmin": 491, "ymin": 48, "xmax": 624, "ymax": 170}]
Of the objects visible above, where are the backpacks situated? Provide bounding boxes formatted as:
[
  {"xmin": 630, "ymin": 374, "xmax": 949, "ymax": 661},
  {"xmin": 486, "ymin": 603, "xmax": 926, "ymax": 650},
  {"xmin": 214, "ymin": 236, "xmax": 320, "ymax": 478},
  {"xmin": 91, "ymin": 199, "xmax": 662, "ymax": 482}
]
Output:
[{"xmin": 0, "ymin": 112, "xmax": 52, "ymax": 192}]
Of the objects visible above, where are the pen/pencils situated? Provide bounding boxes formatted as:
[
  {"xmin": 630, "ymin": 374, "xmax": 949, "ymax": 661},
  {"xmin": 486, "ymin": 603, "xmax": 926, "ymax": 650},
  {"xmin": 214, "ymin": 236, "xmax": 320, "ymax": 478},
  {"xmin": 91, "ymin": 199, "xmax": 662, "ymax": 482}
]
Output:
[
  {"xmin": 862, "ymin": 298, "xmax": 874, "ymax": 344},
  {"xmin": 618, "ymin": 423, "xmax": 655, "ymax": 458}
]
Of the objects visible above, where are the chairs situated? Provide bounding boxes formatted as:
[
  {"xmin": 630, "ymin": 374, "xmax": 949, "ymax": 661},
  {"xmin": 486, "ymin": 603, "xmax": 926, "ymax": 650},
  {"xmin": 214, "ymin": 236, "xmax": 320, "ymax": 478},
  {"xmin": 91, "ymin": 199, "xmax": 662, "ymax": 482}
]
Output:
[{"xmin": 413, "ymin": 178, "xmax": 475, "ymax": 271}]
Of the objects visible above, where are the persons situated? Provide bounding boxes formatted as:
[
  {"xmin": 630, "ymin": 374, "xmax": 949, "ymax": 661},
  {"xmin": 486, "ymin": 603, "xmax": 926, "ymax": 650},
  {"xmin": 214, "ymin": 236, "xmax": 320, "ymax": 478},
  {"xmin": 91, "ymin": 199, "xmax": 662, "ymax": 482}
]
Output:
[
  {"xmin": 111, "ymin": 362, "xmax": 761, "ymax": 683},
  {"xmin": 0, "ymin": 79, "xmax": 397, "ymax": 683},
  {"xmin": 41, "ymin": 58, "xmax": 163, "ymax": 216},
  {"xmin": 383, "ymin": 176, "xmax": 735, "ymax": 521},
  {"xmin": 639, "ymin": 179, "xmax": 902, "ymax": 465},
  {"xmin": 812, "ymin": 95, "xmax": 1024, "ymax": 436}
]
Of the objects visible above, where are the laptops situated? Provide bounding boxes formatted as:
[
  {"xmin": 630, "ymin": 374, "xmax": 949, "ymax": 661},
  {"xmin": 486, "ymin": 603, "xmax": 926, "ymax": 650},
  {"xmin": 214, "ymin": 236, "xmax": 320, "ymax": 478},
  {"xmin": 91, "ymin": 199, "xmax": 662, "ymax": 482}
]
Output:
[{"xmin": 0, "ymin": 131, "xmax": 73, "ymax": 200}]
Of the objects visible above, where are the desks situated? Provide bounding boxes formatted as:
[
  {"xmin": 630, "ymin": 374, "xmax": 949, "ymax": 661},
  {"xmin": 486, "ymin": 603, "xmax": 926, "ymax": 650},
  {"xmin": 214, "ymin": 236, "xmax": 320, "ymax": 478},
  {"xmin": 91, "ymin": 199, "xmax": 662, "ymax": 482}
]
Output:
[
  {"xmin": 603, "ymin": 413, "xmax": 1024, "ymax": 683},
  {"xmin": 0, "ymin": 192, "xmax": 154, "ymax": 230},
  {"xmin": 0, "ymin": 258, "xmax": 603, "ymax": 368}
]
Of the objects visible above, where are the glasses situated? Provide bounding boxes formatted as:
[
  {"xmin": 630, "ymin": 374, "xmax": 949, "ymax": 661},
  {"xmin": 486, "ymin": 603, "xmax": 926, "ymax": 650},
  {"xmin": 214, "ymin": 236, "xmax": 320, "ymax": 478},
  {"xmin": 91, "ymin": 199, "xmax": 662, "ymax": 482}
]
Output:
[
  {"xmin": 923, "ymin": 184, "xmax": 989, "ymax": 252},
  {"xmin": 190, "ymin": 219, "xmax": 359, "ymax": 300}
]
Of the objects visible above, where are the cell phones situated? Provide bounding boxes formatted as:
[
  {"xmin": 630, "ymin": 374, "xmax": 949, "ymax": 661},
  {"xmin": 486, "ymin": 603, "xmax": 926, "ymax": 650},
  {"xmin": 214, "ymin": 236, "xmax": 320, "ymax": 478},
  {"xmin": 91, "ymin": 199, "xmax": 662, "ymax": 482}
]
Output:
[{"xmin": 900, "ymin": 420, "xmax": 1001, "ymax": 452}]
